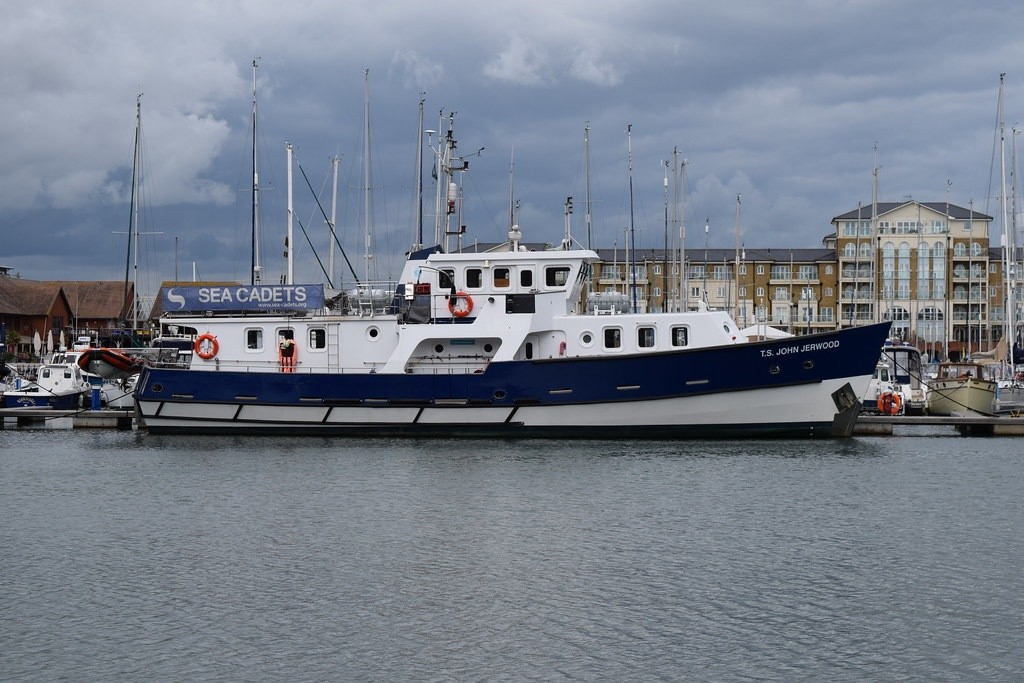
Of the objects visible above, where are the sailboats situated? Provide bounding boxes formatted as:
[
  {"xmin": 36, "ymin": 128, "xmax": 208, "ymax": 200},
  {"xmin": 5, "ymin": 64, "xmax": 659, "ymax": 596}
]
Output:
[{"xmin": 865, "ymin": 70, "xmax": 1022, "ymax": 418}]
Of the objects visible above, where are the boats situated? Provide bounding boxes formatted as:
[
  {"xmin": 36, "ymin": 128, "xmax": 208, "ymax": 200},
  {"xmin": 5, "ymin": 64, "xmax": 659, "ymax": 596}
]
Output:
[
  {"xmin": 142, "ymin": 59, "xmax": 897, "ymax": 440},
  {"xmin": 2, "ymin": 341, "xmax": 140, "ymax": 409}
]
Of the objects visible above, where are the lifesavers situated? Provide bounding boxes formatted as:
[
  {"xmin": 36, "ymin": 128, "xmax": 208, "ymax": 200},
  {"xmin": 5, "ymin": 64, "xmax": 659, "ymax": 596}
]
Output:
[
  {"xmin": 194, "ymin": 334, "xmax": 219, "ymax": 360},
  {"xmin": 878, "ymin": 391, "xmax": 901, "ymax": 414},
  {"xmin": 449, "ymin": 293, "xmax": 474, "ymax": 315}
]
